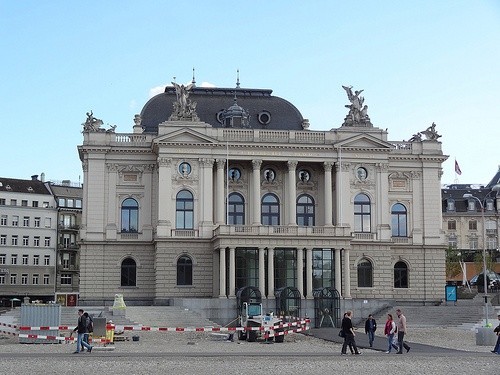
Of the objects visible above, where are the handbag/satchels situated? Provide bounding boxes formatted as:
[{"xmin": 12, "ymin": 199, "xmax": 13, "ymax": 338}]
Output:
[
  {"xmin": 390, "ymin": 321, "xmax": 397, "ymax": 334},
  {"xmin": 338, "ymin": 330, "xmax": 345, "ymax": 337}
]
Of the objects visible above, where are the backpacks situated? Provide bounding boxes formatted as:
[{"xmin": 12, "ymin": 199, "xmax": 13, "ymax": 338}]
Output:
[{"xmin": 86, "ymin": 319, "xmax": 94, "ymax": 332}]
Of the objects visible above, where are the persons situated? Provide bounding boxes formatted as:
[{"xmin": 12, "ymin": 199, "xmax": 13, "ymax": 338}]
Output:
[
  {"xmin": 430, "ymin": 121, "xmax": 438, "ymax": 140},
  {"xmin": 365, "ymin": 314, "xmax": 377, "ymax": 347},
  {"xmin": 340, "ymin": 311, "xmax": 361, "ymax": 356},
  {"xmin": 342, "ymin": 85, "xmax": 365, "ymax": 120},
  {"xmin": 171, "ymin": 82, "xmax": 197, "ymax": 116},
  {"xmin": 384, "ymin": 314, "xmax": 398, "ymax": 354},
  {"xmin": 81, "ymin": 312, "xmax": 92, "ymax": 352},
  {"xmin": 396, "ymin": 309, "xmax": 412, "ymax": 354},
  {"xmin": 491, "ymin": 315, "xmax": 500, "ymax": 353},
  {"xmin": 73, "ymin": 309, "xmax": 92, "ymax": 353},
  {"xmin": 83, "ymin": 109, "xmax": 103, "ymax": 131}
]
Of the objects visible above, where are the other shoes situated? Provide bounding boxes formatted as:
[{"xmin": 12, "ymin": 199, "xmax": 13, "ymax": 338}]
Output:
[
  {"xmin": 80, "ymin": 349, "xmax": 85, "ymax": 352},
  {"xmin": 87, "ymin": 347, "xmax": 92, "ymax": 352},
  {"xmin": 74, "ymin": 352, "xmax": 79, "ymax": 353}
]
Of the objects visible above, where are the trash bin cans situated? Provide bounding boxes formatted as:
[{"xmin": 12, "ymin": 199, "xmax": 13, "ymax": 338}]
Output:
[{"xmin": 445, "ymin": 281, "xmax": 457, "ymax": 302}]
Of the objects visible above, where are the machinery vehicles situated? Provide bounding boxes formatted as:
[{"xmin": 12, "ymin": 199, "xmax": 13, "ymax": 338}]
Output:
[{"xmin": 237, "ymin": 299, "xmax": 285, "ymax": 343}]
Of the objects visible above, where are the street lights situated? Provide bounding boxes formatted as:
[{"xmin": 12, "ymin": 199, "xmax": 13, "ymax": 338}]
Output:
[{"xmin": 462, "ymin": 184, "xmax": 500, "ymax": 326}]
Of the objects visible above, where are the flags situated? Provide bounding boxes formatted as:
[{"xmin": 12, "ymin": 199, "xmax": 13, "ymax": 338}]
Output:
[{"xmin": 455, "ymin": 160, "xmax": 461, "ymax": 175}]
